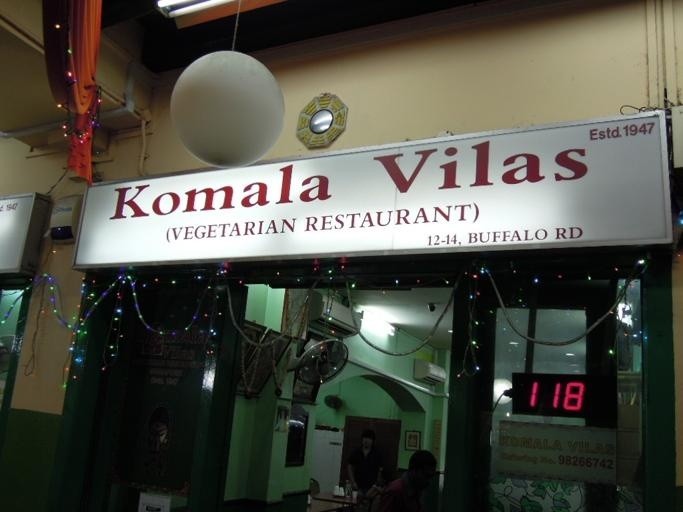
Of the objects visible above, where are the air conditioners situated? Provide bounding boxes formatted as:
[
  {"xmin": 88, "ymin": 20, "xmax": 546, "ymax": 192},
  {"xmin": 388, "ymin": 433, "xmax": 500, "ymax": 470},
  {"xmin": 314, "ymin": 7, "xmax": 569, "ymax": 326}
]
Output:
[
  {"xmin": 414, "ymin": 358, "xmax": 447, "ymax": 387},
  {"xmin": 305, "ymin": 290, "xmax": 360, "ymax": 343}
]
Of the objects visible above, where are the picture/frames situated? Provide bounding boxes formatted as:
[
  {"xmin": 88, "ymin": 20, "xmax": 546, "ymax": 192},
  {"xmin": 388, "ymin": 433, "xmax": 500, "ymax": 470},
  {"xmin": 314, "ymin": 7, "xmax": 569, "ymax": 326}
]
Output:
[
  {"xmin": 291, "ymin": 331, "xmax": 335, "ymax": 405},
  {"xmin": 284, "ymin": 410, "xmax": 309, "ymax": 466},
  {"xmin": 235, "ymin": 319, "xmax": 292, "ymax": 398},
  {"xmin": 337, "ymin": 415, "xmax": 402, "ymax": 492},
  {"xmin": 405, "ymin": 431, "xmax": 421, "ymax": 451}
]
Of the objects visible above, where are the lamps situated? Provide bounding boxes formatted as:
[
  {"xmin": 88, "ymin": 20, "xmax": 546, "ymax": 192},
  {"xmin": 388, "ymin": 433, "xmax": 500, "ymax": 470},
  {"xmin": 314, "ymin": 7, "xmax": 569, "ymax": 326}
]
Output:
[{"xmin": 169, "ymin": 1, "xmax": 285, "ymax": 170}]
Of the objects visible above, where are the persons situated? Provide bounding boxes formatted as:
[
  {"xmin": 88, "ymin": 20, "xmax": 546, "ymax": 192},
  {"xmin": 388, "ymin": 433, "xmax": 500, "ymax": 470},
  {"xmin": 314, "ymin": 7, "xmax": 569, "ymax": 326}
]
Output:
[
  {"xmin": 371, "ymin": 450, "xmax": 439, "ymax": 512},
  {"xmin": 348, "ymin": 428, "xmax": 377, "ymax": 494}
]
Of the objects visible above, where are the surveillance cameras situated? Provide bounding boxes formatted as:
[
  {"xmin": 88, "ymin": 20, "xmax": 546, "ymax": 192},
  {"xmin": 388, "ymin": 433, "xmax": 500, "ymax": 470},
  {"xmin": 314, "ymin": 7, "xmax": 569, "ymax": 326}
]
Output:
[{"xmin": 427, "ymin": 303, "xmax": 437, "ymax": 312}]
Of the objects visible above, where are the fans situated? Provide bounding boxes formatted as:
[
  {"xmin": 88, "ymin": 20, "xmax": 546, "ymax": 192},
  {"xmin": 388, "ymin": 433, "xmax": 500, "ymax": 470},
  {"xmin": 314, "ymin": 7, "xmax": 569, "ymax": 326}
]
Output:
[
  {"xmin": 324, "ymin": 395, "xmax": 343, "ymax": 413},
  {"xmin": 285, "ymin": 338, "xmax": 349, "ymax": 386}
]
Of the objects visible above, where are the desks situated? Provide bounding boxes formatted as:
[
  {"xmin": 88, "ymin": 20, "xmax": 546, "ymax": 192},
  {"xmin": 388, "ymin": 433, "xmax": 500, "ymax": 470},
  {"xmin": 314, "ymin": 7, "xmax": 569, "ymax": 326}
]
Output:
[{"xmin": 305, "ymin": 490, "xmax": 371, "ymax": 512}]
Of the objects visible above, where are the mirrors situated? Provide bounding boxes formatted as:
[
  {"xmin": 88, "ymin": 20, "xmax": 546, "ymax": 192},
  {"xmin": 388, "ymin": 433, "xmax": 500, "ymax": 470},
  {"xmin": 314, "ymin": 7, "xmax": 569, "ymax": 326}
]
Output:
[{"xmin": 295, "ymin": 93, "xmax": 348, "ymax": 150}]
